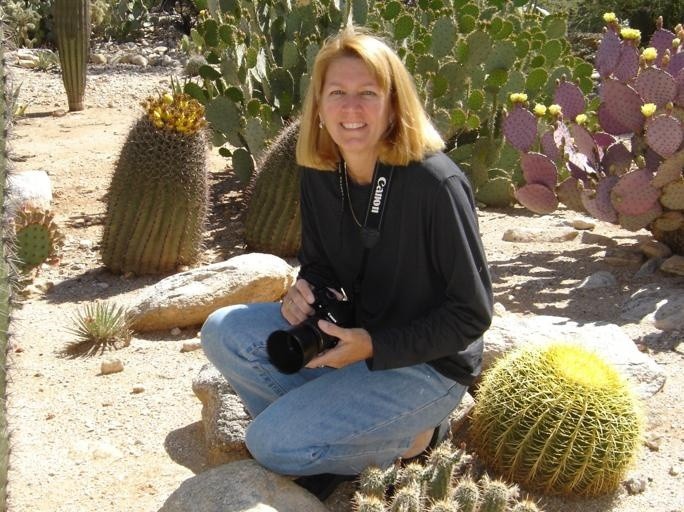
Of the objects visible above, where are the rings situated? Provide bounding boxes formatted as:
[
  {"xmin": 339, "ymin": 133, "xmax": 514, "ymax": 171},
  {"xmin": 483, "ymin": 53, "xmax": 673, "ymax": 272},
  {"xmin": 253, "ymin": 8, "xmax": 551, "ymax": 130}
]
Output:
[
  {"xmin": 320, "ymin": 364, "xmax": 325, "ymax": 368},
  {"xmin": 287, "ymin": 299, "xmax": 291, "ymax": 304}
]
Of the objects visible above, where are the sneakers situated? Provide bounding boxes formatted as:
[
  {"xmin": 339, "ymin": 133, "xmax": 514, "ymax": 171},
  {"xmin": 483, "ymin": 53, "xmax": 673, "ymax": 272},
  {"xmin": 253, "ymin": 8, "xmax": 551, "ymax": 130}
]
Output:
[
  {"xmin": 399, "ymin": 419, "xmax": 451, "ymax": 469},
  {"xmin": 293, "ymin": 473, "xmax": 338, "ymax": 502}
]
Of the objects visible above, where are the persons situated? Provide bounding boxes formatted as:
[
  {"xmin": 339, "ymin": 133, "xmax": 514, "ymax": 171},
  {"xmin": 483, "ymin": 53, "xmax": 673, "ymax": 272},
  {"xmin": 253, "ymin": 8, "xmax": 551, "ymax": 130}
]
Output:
[{"xmin": 201, "ymin": 32, "xmax": 495, "ymax": 477}]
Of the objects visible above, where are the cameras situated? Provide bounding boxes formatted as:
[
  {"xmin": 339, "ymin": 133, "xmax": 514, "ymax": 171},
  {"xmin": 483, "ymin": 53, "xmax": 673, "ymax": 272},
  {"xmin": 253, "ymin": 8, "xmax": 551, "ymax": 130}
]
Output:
[{"xmin": 265, "ymin": 287, "xmax": 351, "ymax": 374}]
